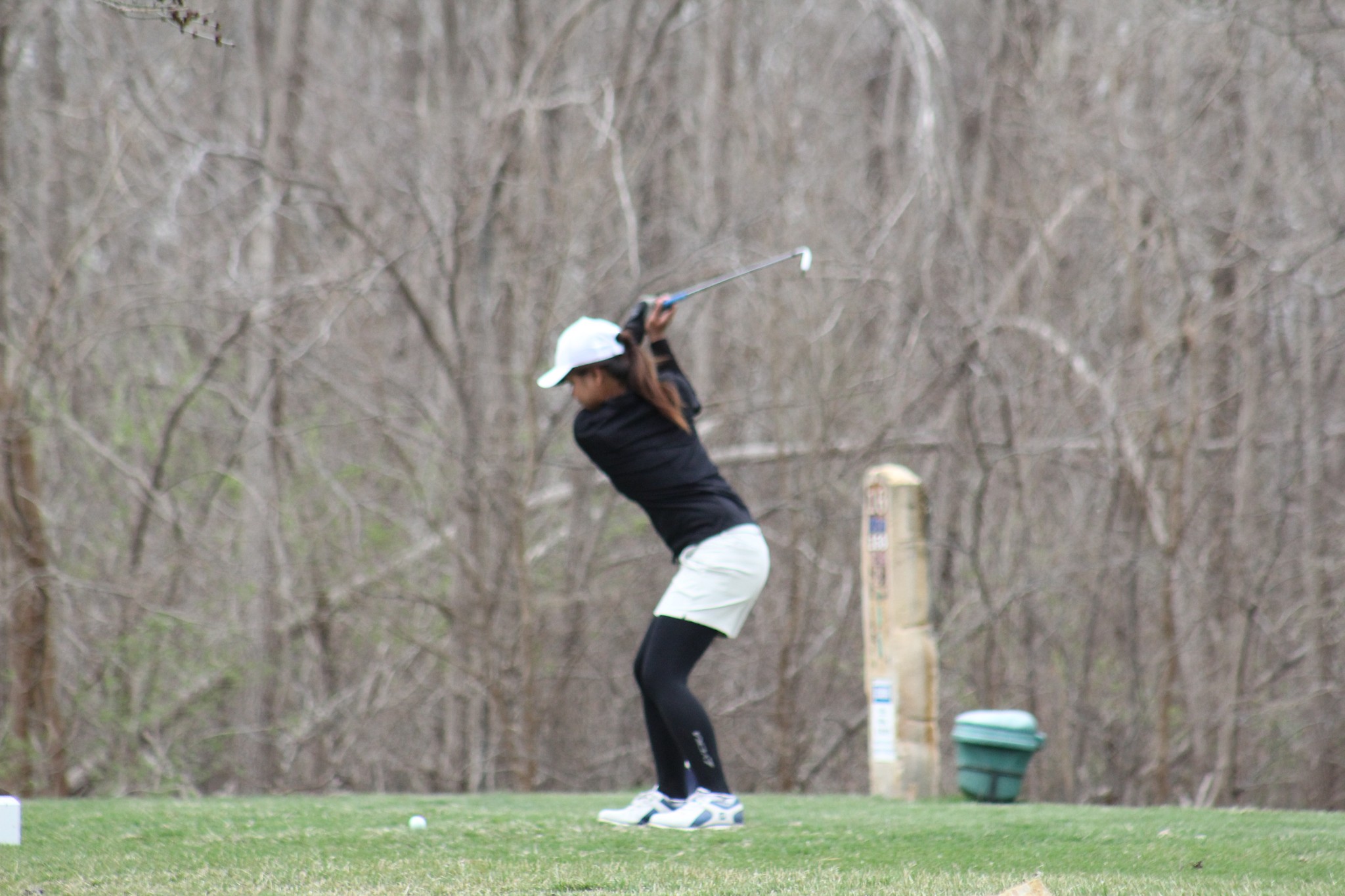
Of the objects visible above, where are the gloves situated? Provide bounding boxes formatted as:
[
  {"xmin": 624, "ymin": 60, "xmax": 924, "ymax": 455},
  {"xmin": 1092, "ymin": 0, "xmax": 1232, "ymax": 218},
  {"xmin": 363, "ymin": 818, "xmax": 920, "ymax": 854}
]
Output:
[{"xmin": 623, "ymin": 302, "xmax": 647, "ymax": 346}]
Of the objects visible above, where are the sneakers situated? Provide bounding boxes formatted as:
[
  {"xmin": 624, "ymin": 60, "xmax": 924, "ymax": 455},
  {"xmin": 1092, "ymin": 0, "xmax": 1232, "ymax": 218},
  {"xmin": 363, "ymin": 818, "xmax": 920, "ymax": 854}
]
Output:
[
  {"xmin": 597, "ymin": 785, "xmax": 686, "ymax": 827},
  {"xmin": 646, "ymin": 787, "xmax": 745, "ymax": 831}
]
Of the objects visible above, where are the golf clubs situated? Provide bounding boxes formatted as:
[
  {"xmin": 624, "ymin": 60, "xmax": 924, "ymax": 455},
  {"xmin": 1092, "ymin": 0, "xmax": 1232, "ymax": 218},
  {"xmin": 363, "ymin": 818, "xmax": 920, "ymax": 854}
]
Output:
[{"xmin": 658, "ymin": 244, "xmax": 814, "ymax": 312}]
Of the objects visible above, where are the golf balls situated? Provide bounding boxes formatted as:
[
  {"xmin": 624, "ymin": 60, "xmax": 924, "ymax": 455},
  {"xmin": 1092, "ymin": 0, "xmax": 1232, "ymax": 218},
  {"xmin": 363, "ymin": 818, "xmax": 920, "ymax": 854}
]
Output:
[{"xmin": 408, "ymin": 815, "xmax": 427, "ymax": 830}]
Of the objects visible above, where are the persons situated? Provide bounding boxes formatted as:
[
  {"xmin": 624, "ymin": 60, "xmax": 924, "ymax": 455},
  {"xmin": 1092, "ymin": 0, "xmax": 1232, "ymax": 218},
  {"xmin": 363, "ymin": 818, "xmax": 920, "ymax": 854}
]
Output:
[{"xmin": 536, "ymin": 294, "xmax": 770, "ymax": 833}]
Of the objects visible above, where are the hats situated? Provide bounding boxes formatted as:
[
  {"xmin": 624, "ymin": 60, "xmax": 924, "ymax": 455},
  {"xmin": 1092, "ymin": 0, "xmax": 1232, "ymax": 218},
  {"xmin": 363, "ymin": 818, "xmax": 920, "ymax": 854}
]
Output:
[{"xmin": 537, "ymin": 316, "xmax": 626, "ymax": 388}]
